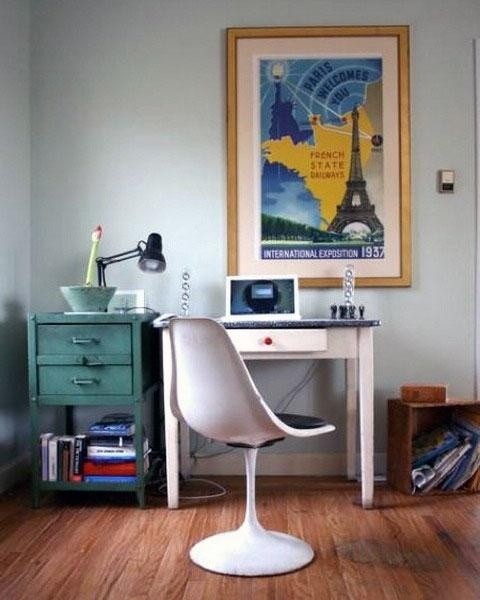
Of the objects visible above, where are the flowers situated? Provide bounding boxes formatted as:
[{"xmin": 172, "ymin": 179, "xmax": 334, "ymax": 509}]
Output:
[{"xmin": 85, "ymin": 226, "xmax": 102, "ymax": 286}]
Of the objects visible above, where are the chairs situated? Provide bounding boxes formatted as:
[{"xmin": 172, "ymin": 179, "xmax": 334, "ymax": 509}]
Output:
[{"xmin": 169, "ymin": 316, "xmax": 337, "ymax": 577}]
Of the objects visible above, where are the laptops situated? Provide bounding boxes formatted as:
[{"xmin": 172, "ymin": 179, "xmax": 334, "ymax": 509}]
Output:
[{"xmin": 221, "ymin": 274, "xmax": 303, "ymax": 322}]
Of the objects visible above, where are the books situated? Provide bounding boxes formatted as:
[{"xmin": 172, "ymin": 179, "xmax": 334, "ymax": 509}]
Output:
[
  {"xmin": 410, "ymin": 420, "xmax": 480, "ymax": 496},
  {"xmin": 39, "ymin": 413, "xmax": 148, "ymax": 487}
]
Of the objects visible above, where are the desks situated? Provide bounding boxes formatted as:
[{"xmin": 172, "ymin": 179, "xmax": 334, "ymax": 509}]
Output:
[{"xmin": 155, "ymin": 316, "xmax": 380, "ymax": 509}]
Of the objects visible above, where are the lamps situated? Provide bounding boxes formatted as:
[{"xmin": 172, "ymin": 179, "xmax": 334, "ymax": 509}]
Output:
[{"xmin": 96, "ymin": 234, "xmax": 167, "ymax": 313}]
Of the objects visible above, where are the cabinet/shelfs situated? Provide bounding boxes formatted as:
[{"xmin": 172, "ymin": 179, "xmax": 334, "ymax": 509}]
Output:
[
  {"xmin": 25, "ymin": 312, "xmax": 166, "ymax": 508},
  {"xmin": 387, "ymin": 398, "xmax": 480, "ymax": 497}
]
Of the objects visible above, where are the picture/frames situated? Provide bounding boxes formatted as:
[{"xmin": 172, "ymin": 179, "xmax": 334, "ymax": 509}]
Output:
[{"xmin": 226, "ymin": 25, "xmax": 413, "ymax": 288}]
